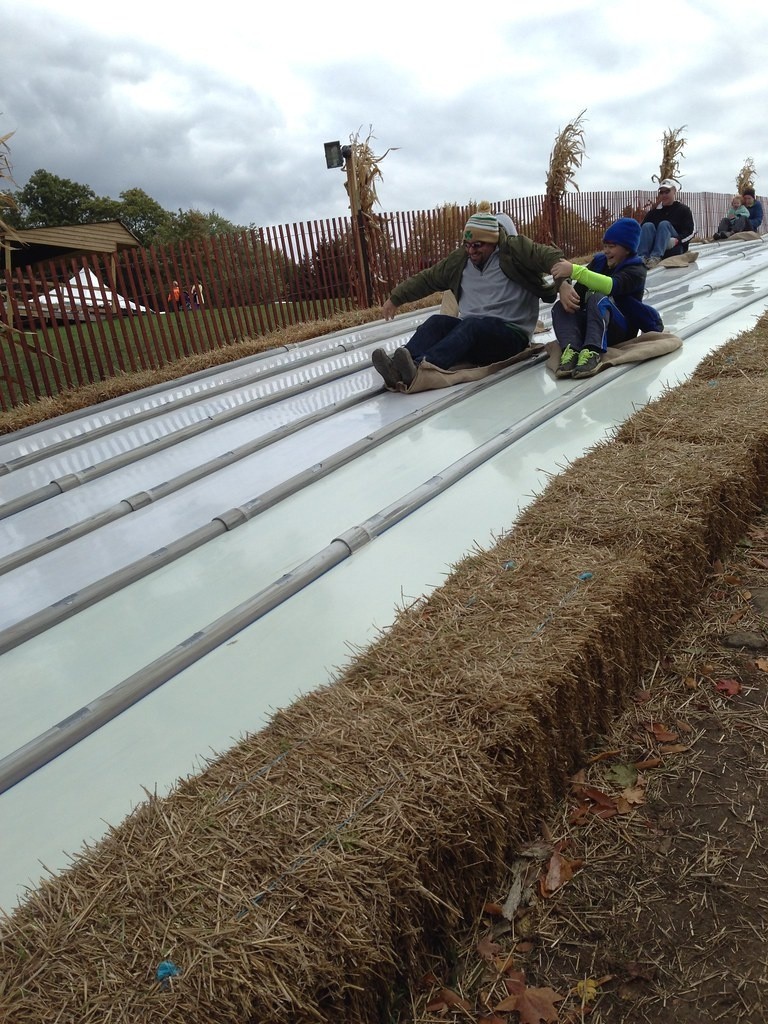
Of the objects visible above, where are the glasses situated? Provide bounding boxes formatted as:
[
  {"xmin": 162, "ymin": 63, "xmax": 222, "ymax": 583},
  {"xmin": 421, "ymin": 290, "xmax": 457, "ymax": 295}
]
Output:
[
  {"xmin": 463, "ymin": 241, "xmax": 485, "ymax": 251},
  {"xmin": 659, "ymin": 189, "xmax": 673, "ymax": 195}
]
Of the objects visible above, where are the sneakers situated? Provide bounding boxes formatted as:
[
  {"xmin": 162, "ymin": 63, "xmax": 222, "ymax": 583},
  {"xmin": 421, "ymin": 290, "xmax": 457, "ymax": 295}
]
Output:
[
  {"xmin": 573, "ymin": 348, "xmax": 605, "ymax": 379},
  {"xmin": 554, "ymin": 343, "xmax": 580, "ymax": 379}
]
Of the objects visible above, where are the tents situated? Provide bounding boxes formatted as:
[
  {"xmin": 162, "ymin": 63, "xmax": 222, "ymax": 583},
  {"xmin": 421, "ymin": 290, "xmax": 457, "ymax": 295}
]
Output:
[{"xmin": 23, "ymin": 266, "xmax": 147, "ymax": 311}]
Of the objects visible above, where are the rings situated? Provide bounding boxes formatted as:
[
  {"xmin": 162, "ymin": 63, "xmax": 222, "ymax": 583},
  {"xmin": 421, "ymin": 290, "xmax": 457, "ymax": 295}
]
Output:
[{"xmin": 572, "ymin": 298, "xmax": 574, "ymax": 301}]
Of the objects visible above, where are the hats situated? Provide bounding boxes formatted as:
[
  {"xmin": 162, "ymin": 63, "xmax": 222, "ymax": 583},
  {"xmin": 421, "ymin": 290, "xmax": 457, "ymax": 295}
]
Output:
[
  {"xmin": 658, "ymin": 178, "xmax": 678, "ymax": 193},
  {"xmin": 742, "ymin": 187, "xmax": 756, "ymax": 199},
  {"xmin": 461, "ymin": 201, "xmax": 500, "ymax": 244},
  {"xmin": 603, "ymin": 217, "xmax": 643, "ymax": 253}
]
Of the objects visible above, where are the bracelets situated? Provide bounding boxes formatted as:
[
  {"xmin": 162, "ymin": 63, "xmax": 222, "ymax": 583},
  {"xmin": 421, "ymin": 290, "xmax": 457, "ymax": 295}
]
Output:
[{"xmin": 675, "ymin": 239, "xmax": 678, "ymax": 245}]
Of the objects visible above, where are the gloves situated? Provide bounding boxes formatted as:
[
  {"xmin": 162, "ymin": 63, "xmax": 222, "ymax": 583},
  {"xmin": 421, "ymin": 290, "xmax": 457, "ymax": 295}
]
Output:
[{"xmin": 667, "ymin": 237, "xmax": 678, "ymax": 250}]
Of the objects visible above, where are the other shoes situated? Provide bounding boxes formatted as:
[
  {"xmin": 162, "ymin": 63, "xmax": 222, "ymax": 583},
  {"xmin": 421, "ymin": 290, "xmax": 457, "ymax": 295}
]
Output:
[
  {"xmin": 644, "ymin": 258, "xmax": 662, "ymax": 269},
  {"xmin": 394, "ymin": 346, "xmax": 419, "ymax": 391},
  {"xmin": 371, "ymin": 348, "xmax": 403, "ymax": 390},
  {"xmin": 713, "ymin": 230, "xmax": 732, "ymax": 241}
]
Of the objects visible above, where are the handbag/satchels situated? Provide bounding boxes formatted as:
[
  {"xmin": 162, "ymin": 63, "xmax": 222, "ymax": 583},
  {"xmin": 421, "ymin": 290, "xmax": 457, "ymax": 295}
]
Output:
[{"xmin": 196, "ymin": 294, "xmax": 205, "ymax": 305}]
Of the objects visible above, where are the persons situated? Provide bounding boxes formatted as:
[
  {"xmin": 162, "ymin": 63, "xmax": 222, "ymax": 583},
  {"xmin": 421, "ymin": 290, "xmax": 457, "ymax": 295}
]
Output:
[
  {"xmin": 713, "ymin": 189, "xmax": 763, "ymax": 240},
  {"xmin": 168, "ymin": 281, "xmax": 180, "ymax": 312},
  {"xmin": 551, "ymin": 218, "xmax": 664, "ymax": 378},
  {"xmin": 372, "ymin": 202, "xmax": 580, "ymax": 392},
  {"xmin": 638, "ymin": 179, "xmax": 696, "ymax": 268},
  {"xmin": 726, "ymin": 196, "xmax": 750, "ymax": 225},
  {"xmin": 191, "ymin": 279, "xmax": 204, "ymax": 305}
]
